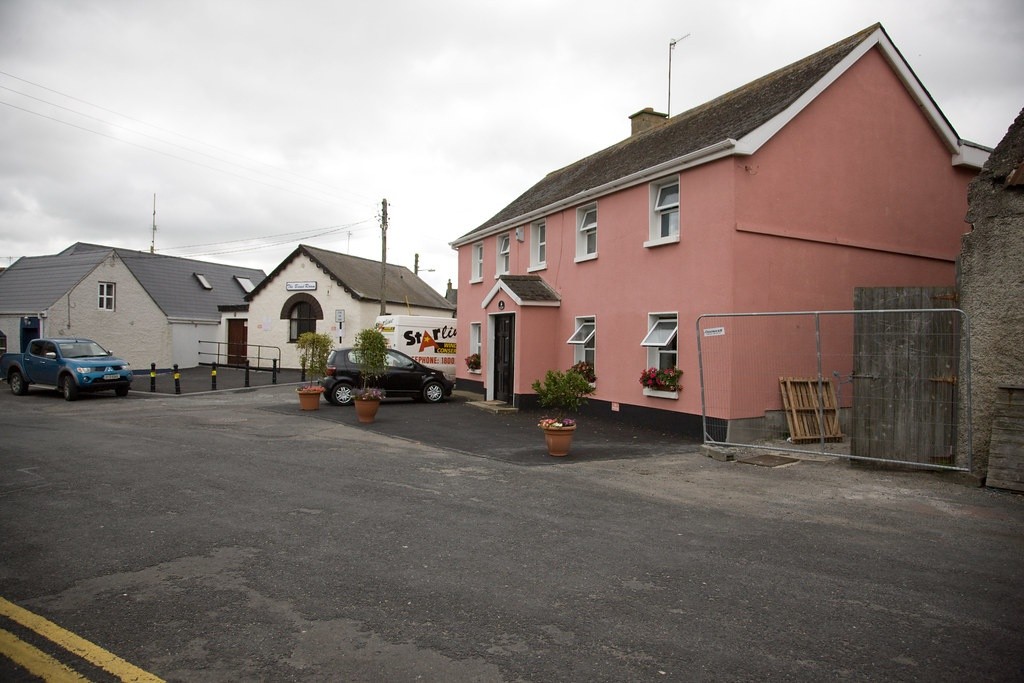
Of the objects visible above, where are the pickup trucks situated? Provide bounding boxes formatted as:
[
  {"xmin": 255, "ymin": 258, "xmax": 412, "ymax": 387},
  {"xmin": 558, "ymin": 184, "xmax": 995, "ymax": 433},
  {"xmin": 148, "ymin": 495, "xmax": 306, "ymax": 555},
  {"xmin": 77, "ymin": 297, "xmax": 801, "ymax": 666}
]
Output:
[{"xmin": 0, "ymin": 337, "xmax": 134, "ymax": 401}]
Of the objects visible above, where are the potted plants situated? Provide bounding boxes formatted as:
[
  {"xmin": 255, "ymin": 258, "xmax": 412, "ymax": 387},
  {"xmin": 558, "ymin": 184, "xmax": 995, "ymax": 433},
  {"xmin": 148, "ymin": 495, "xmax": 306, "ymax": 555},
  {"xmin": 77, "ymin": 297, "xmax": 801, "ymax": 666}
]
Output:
[
  {"xmin": 352, "ymin": 328, "xmax": 389, "ymax": 423},
  {"xmin": 531, "ymin": 370, "xmax": 597, "ymax": 455},
  {"xmin": 293, "ymin": 332, "xmax": 336, "ymax": 410}
]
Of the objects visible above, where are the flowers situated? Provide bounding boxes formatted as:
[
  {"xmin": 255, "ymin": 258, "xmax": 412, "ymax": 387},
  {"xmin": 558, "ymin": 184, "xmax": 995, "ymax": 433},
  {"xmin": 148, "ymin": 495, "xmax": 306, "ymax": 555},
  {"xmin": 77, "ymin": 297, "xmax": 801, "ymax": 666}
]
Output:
[
  {"xmin": 637, "ymin": 367, "xmax": 685, "ymax": 392},
  {"xmin": 566, "ymin": 360, "xmax": 598, "ymax": 382},
  {"xmin": 465, "ymin": 353, "xmax": 481, "ymax": 371}
]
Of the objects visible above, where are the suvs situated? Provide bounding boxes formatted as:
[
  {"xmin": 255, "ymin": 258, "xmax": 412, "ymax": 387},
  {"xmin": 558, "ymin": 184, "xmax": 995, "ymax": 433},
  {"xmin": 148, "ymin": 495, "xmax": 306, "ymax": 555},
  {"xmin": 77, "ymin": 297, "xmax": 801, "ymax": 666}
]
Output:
[{"xmin": 320, "ymin": 346, "xmax": 454, "ymax": 406}]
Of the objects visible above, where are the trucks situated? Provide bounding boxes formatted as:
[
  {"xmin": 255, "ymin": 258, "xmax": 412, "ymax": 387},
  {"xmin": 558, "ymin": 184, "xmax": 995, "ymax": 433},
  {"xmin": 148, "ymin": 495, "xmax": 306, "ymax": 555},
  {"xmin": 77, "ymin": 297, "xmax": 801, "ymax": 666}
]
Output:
[{"xmin": 374, "ymin": 315, "xmax": 457, "ymax": 390}]
